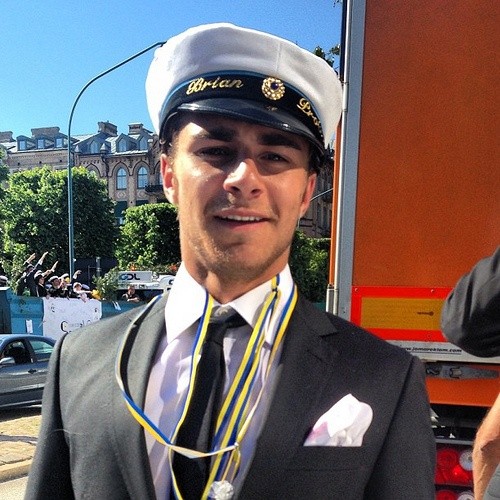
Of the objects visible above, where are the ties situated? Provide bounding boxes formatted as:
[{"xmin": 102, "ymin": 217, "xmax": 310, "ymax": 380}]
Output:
[{"xmin": 168, "ymin": 306, "xmax": 249, "ymax": 500}]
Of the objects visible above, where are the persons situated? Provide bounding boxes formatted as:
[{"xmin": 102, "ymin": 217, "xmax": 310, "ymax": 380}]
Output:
[
  {"xmin": 119, "ymin": 286, "xmax": 141, "ymax": 302},
  {"xmin": 16, "ymin": 251, "xmax": 102, "ymax": 301},
  {"xmin": 26, "ymin": 23, "xmax": 436, "ymax": 500},
  {"xmin": 441, "ymin": 244, "xmax": 500, "ymax": 500}
]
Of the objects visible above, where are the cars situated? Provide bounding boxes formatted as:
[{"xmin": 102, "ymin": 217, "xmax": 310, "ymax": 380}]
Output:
[{"xmin": 1, "ymin": 333, "xmax": 61, "ymax": 409}]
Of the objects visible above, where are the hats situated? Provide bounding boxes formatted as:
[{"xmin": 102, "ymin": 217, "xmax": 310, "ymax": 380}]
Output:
[{"xmin": 146, "ymin": 22, "xmax": 344, "ymax": 174}]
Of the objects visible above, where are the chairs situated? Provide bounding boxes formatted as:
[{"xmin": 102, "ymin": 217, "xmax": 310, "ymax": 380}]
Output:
[{"xmin": 3, "ymin": 347, "xmax": 26, "ymax": 364}]
